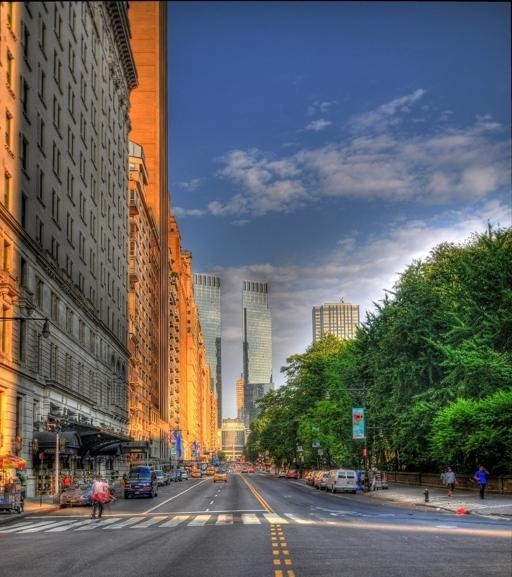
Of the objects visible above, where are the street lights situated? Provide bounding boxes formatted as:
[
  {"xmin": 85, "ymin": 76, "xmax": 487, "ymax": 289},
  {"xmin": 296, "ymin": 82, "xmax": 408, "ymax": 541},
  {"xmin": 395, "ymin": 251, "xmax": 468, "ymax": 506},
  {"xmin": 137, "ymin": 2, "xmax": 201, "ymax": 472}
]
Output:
[
  {"xmin": 322, "ymin": 383, "xmax": 371, "ymax": 492},
  {"xmin": 0, "ymin": 317, "xmax": 51, "ymax": 339},
  {"xmin": 169, "ymin": 429, "xmax": 190, "ymax": 471}
]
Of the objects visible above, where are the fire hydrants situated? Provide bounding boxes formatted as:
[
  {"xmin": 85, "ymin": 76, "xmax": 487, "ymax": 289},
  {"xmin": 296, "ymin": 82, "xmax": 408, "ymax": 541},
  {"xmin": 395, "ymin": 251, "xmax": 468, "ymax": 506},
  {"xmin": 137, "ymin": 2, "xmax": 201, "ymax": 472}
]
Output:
[{"xmin": 423, "ymin": 488, "xmax": 430, "ymax": 502}]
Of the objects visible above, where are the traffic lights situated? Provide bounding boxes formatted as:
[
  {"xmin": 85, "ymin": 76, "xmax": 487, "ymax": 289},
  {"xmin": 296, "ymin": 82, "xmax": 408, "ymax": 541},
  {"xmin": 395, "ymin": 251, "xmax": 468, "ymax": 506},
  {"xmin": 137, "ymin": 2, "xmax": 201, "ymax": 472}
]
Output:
[
  {"xmin": 47, "ymin": 423, "xmax": 56, "ymax": 432},
  {"xmin": 54, "ymin": 417, "xmax": 61, "ymax": 432}
]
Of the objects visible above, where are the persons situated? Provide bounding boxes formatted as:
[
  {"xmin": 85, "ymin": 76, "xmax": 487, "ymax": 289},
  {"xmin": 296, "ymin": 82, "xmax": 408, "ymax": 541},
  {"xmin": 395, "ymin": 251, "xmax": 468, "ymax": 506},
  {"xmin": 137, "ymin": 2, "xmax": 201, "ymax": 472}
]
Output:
[
  {"xmin": 474, "ymin": 465, "xmax": 490, "ymax": 500},
  {"xmin": 440, "ymin": 469, "xmax": 447, "ymax": 484},
  {"xmin": 445, "ymin": 467, "xmax": 458, "ymax": 496},
  {"xmin": 91, "ymin": 477, "xmax": 115, "ymax": 520}
]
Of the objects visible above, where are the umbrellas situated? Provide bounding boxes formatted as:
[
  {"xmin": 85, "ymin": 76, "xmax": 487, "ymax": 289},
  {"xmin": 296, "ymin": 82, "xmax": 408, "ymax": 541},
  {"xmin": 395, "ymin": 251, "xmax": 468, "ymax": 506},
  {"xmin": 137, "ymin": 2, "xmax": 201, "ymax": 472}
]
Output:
[{"xmin": 0, "ymin": 455, "xmax": 27, "ymax": 485}]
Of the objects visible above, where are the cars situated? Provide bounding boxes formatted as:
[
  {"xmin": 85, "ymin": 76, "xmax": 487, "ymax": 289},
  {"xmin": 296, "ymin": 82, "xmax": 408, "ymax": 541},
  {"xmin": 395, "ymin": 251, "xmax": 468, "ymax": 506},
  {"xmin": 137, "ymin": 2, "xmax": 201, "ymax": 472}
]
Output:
[
  {"xmin": 151, "ymin": 461, "xmax": 389, "ymax": 490},
  {"xmin": 59, "ymin": 482, "xmax": 115, "ymax": 508}
]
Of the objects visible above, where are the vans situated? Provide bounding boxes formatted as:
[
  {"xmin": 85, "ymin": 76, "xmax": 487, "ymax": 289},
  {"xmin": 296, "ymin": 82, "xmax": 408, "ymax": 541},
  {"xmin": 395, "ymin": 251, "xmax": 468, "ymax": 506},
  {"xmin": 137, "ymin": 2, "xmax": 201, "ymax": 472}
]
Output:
[
  {"xmin": 122, "ymin": 466, "xmax": 159, "ymax": 500},
  {"xmin": 325, "ymin": 468, "xmax": 360, "ymax": 495}
]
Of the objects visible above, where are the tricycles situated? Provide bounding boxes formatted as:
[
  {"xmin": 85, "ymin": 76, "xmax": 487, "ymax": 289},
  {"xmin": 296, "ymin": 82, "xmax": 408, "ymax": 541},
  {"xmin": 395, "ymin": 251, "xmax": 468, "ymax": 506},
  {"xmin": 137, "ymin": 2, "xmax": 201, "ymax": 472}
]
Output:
[{"xmin": 0, "ymin": 484, "xmax": 27, "ymax": 514}]
